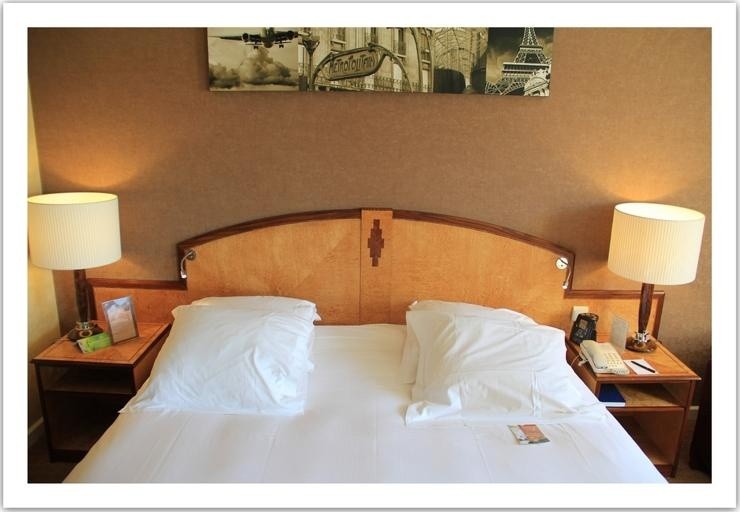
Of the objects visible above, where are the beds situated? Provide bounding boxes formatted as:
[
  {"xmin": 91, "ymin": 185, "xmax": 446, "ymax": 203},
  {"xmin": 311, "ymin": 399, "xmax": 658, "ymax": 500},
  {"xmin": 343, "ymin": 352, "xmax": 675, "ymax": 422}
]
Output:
[{"xmin": 60, "ymin": 206, "xmax": 666, "ymax": 482}]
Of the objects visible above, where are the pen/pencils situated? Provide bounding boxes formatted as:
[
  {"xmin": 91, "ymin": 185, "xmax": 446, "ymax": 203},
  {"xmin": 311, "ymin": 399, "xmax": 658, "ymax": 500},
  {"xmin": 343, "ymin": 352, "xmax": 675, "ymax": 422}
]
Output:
[{"xmin": 631, "ymin": 361, "xmax": 655, "ymax": 373}]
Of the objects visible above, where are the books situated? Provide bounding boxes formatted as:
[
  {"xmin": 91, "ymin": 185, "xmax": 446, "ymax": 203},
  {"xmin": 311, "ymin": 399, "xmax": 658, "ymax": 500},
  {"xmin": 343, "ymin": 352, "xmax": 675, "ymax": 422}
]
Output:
[{"xmin": 596, "ymin": 382, "xmax": 627, "ymax": 409}]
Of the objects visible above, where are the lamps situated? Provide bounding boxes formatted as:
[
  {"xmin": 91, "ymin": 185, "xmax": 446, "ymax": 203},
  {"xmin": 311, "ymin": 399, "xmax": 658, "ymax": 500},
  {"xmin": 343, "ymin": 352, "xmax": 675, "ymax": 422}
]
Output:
[
  {"xmin": 27, "ymin": 192, "xmax": 122, "ymax": 341},
  {"xmin": 607, "ymin": 202, "xmax": 706, "ymax": 353}
]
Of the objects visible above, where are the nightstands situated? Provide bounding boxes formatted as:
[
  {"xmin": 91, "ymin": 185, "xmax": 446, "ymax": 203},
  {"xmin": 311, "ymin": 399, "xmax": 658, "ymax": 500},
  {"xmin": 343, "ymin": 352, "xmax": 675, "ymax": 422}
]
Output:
[
  {"xmin": 31, "ymin": 319, "xmax": 172, "ymax": 460},
  {"xmin": 565, "ymin": 331, "xmax": 703, "ymax": 479}
]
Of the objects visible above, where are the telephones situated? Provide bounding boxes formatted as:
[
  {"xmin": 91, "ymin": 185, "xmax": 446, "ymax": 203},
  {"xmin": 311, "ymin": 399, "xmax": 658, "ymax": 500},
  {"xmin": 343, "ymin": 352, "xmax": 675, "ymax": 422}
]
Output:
[{"xmin": 579, "ymin": 340, "xmax": 630, "ymax": 374}]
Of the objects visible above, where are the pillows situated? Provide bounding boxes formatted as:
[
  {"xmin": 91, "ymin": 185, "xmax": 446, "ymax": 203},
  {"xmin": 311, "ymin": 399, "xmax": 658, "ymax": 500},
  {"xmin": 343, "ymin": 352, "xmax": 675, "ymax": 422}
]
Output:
[
  {"xmin": 116, "ymin": 294, "xmax": 321, "ymax": 418},
  {"xmin": 398, "ymin": 300, "xmax": 600, "ymax": 426}
]
